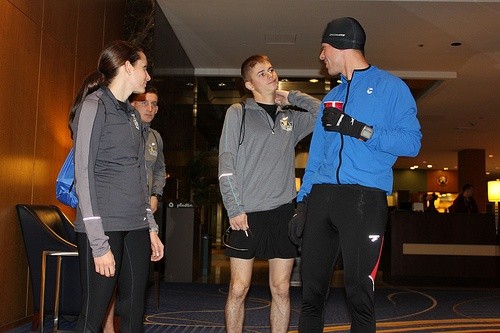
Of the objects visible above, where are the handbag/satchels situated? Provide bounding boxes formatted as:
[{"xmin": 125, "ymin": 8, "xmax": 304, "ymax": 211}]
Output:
[{"xmin": 55, "ymin": 146, "xmax": 80, "ymax": 208}]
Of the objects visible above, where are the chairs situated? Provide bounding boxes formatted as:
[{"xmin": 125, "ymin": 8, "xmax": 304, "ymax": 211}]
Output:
[{"xmin": 15, "ymin": 204, "xmax": 121, "ymax": 333}]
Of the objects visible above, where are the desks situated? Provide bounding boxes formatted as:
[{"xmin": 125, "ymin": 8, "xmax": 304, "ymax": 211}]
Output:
[{"xmin": 39, "ymin": 251, "xmax": 78, "ymax": 333}]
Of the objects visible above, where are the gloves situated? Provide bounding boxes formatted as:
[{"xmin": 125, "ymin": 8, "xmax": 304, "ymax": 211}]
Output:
[
  {"xmin": 288, "ymin": 202, "xmax": 307, "ymax": 248},
  {"xmin": 320, "ymin": 107, "xmax": 367, "ymax": 139}
]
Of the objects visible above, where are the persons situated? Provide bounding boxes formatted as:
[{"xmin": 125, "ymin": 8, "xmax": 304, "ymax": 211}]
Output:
[
  {"xmin": 130, "ymin": 84, "xmax": 166, "ymax": 212},
  {"xmin": 290, "ymin": 17, "xmax": 422, "ymax": 333},
  {"xmin": 453, "ymin": 184, "xmax": 479, "ymax": 214},
  {"xmin": 68, "ymin": 41, "xmax": 165, "ymax": 333},
  {"xmin": 217, "ymin": 55, "xmax": 323, "ymax": 333},
  {"xmin": 54, "ymin": 147, "xmax": 116, "ymax": 333}
]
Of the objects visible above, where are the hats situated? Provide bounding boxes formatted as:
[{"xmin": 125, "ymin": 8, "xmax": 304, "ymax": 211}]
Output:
[{"xmin": 321, "ymin": 17, "xmax": 366, "ymax": 50}]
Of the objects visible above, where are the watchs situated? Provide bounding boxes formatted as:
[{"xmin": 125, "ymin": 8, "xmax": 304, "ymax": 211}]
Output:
[{"xmin": 151, "ymin": 193, "xmax": 162, "ymax": 200}]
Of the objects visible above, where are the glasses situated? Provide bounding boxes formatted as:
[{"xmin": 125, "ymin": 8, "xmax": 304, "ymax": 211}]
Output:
[
  {"xmin": 222, "ymin": 225, "xmax": 254, "ymax": 251},
  {"xmin": 136, "ymin": 101, "xmax": 158, "ymax": 107}
]
